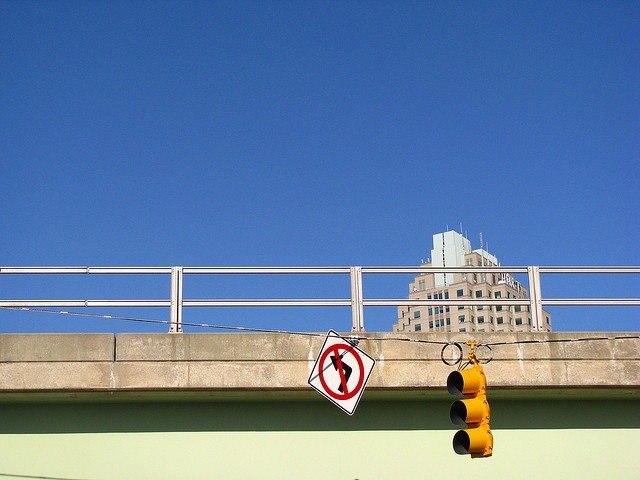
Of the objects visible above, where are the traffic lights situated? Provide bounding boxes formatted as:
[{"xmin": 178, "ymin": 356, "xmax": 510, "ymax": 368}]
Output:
[{"xmin": 447, "ymin": 364, "xmax": 493, "ymax": 458}]
